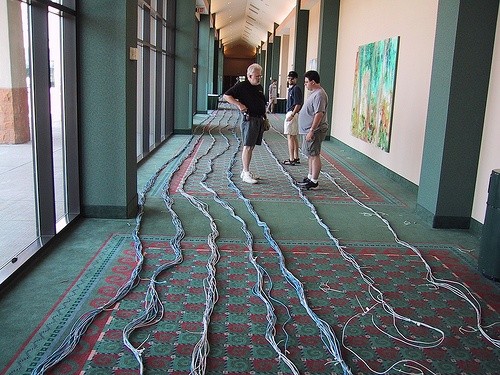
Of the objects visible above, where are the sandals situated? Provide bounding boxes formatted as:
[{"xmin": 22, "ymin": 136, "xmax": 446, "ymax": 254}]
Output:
[
  {"xmin": 289, "ymin": 158, "xmax": 300, "ymax": 164},
  {"xmin": 282, "ymin": 160, "xmax": 295, "ymax": 165}
]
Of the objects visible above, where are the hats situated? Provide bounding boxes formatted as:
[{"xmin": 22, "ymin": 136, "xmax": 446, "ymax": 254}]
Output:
[{"xmin": 288, "ymin": 71, "xmax": 298, "ymax": 77}]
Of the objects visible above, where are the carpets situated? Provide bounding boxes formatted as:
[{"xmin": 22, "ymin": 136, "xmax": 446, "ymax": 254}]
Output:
[
  {"xmin": 153, "ymin": 138, "xmax": 408, "ymax": 208},
  {"xmin": 204, "ymin": 102, "xmax": 285, "ymax": 134},
  {"xmin": 0, "ymin": 232, "xmax": 500, "ymax": 375}
]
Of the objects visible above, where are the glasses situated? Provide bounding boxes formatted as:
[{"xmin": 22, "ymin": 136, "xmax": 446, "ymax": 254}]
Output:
[{"xmin": 256, "ymin": 75, "xmax": 262, "ymax": 78}]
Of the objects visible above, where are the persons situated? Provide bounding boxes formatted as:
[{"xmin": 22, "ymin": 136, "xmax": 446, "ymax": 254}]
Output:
[
  {"xmin": 222, "ymin": 63, "xmax": 271, "ymax": 185},
  {"xmin": 296, "ymin": 70, "xmax": 330, "ymax": 190},
  {"xmin": 265, "ymin": 79, "xmax": 279, "ymax": 114},
  {"xmin": 281, "ymin": 70, "xmax": 303, "ymax": 166}
]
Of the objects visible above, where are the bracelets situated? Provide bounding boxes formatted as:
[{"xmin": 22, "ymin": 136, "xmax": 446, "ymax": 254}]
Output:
[
  {"xmin": 291, "ymin": 114, "xmax": 294, "ymax": 117},
  {"xmin": 310, "ymin": 128, "xmax": 314, "ymax": 133}
]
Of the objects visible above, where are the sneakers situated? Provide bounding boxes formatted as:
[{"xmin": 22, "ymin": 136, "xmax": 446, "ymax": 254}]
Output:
[
  {"xmin": 240, "ymin": 169, "xmax": 259, "ymax": 179},
  {"xmin": 297, "ymin": 177, "xmax": 311, "ymax": 185},
  {"xmin": 243, "ymin": 172, "xmax": 258, "ymax": 184},
  {"xmin": 301, "ymin": 181, "xmax": 319, "ymax": 190}
]
